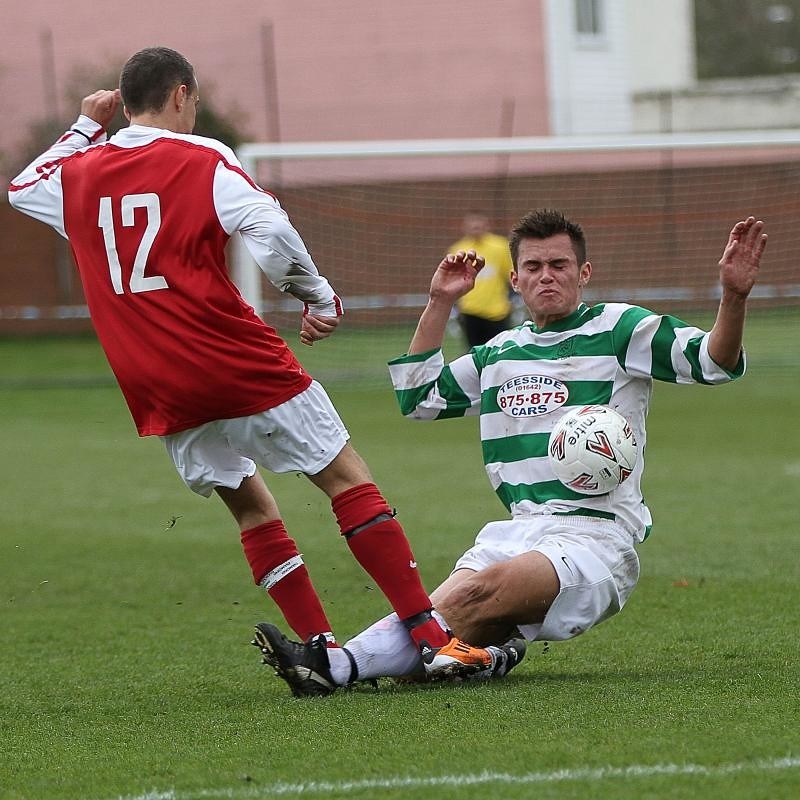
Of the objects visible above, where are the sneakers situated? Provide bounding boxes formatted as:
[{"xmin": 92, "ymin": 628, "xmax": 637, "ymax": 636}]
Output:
[
  {"xmin": 417, "ymin": 635, "xmax": 491, "ymax": 683},
  {"xmin": 488, "ymin": 637, "xmax": 528, "ymax": 678},
  {"xmin": 250, "ymin": 623, "xmax": 336, "ymax": 699}
]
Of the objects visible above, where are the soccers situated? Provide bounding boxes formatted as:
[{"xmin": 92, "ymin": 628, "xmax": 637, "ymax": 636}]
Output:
[{"xmin": 549, "ymin": 406, "xmax": 639, "ymax": 497}]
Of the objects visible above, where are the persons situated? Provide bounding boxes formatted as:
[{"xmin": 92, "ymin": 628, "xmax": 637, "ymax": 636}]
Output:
[
  {"xmin": 245, "ymin": 202, "xmax": 771, "ymax": 697},
  {"xmin": 438, "ymin": 205, "xmax": 521, "ymax": 358},
  {"xmin": 8, "ymin": 44, "xmax": 526, "ymax": 692}
]
